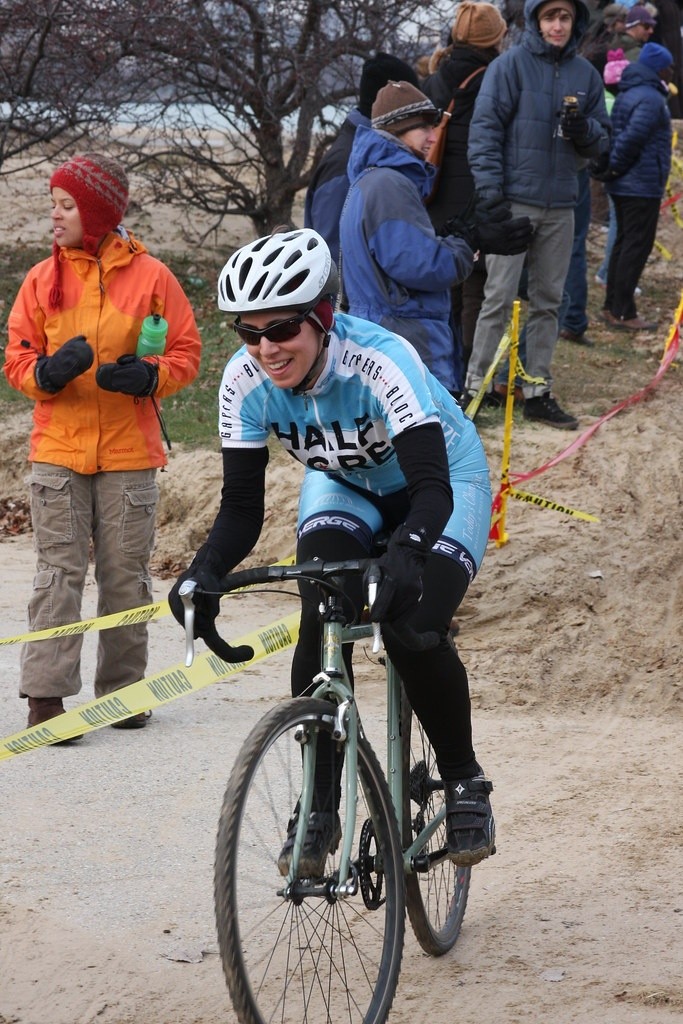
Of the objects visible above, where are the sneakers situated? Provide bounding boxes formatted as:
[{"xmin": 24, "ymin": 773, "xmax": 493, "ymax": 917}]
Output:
[
  {"xmin": 461, "ymin": 383, "xmax": 580, "ymax": 429},
  {"xmin": 444, "ymin": 761, "xmax": 495, "ymax": 867},
  {"xmin": 277, "ymin": 808, "xmax": 343, "ymax": 879},
  {"xmin": 534, "ymin": 0, "xmax": 676, "ymax": 87}
]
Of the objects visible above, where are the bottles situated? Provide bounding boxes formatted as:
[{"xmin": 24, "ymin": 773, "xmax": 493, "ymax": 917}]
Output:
[{"xmin": 135, "ymin": 313, "xmax": 168, "ymax": 362}]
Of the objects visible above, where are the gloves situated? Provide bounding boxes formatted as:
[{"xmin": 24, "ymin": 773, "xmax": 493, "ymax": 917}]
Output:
[
  {"xmin": 33, "ymin": 334, "xmax": 95, "ymax": 392},
  {"xmin": 459, "ymin": 216, "xmax": 534, "ymax": 255},
  {"xmin": 469, "ymin": 195, "xmax": 512, "ymax": 225},
  {"xmin": 556, "ymin": 109, "xmax": 588, "ymax": 138},
  {"xmin": 95, "ymin": 353, "xmax": 159, "ymax": 397},
  {"xmin": 168, "ymin": 543, "xmax": 226, "ymax": 640},
  {"xmin": 361, "ymin": 526, "xmax": 429, "ymax": 623}
]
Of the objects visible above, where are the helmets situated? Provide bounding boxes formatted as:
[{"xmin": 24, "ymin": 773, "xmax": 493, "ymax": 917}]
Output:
[{"xmin": 218, "ymin": 227, "xmax": 340, "ymax": 312}]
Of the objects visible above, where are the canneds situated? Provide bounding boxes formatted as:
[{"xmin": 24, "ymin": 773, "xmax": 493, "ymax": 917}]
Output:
[{"xmin": 557, "ymin": 96, "xmax": 579, "ymax": 140}]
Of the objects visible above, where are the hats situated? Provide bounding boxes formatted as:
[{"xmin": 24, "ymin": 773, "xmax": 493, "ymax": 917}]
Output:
[
  {"xmin": 49, "ymin": 152, "xmax": 128, "ymax": 309},
  {"xmin": 359, "ymin": 51, "xmax": 421, "ymax": 118},
  {"xmin": 452, "ymin": 0, "xmax": 508, "ymax": 48},
  {"xmin": 370, "ymin": 80, "xmax": 439, "ymax": 135}
]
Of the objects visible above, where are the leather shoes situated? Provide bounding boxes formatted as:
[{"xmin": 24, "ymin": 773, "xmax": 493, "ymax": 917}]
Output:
[
  {"xmin": 560, "ymin": 329, "xmax": 593, "ymax": 348},
  {"xmin": 599, "ymin": 308, "xmax": 657, "ymax": 332}
]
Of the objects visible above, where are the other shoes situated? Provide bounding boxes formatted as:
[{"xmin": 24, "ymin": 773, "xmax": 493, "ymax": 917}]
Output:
[
  {"xmin": 111, "ymin": 711, "xmax": 147, "ymax": 730},
  {"xmin": 595, "ymin": 274, "xmax": 642, "ymax": 296},
  {"xmin": 25, "ymin": 698, "xmax": 84, "ymax": 741}
]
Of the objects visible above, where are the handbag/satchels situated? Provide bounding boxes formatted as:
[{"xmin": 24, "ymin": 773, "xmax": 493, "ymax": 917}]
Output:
[{"xmin": 425, "ymin": 111, "xmax": 452, "ymax": 168}]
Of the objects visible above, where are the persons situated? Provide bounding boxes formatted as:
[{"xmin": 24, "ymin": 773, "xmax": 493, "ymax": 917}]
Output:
[
  {"xmin": 5, "ymin": 152, "xmax": 201, "ymax": 747},
  {"xmin": 339, "ymin": 80, "xmax": 537, "ymax": 406},
  {"xmin": 460, "ymin": 0, "xmax": 614, "ymax": 430},
  {"xmin": 421, "ymin": 0, "xmax": 675, "ymax": 404},
  {"xmin": 310, "ymin": 51, "xmax": 421, "ymax": 264},
  {"xmin": 169, "ymin": 226, "xmax": 495, "ymax": 878}
]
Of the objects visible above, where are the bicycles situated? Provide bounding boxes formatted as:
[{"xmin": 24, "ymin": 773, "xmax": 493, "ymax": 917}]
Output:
[{"xmin": 174, "ymin": 532, "xmax": 474, "ymax": 1024}]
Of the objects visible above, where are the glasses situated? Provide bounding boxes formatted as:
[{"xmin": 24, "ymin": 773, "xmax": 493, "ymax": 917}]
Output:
[
  {"xmin": 419, "ymin": 108, "xmax": 443, "ymax": 128},
  {"xmin": 233, "ymin": 309, "xmax": 313, "ymax": 346}
]
